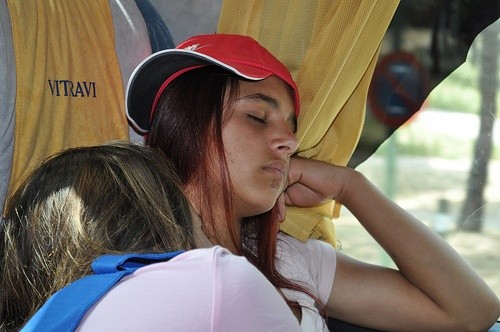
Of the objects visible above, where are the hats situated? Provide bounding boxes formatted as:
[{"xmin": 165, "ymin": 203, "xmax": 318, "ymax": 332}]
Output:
[{"xmin": 124, "ymin": 32, "xmax": 299, "ymax": 141}]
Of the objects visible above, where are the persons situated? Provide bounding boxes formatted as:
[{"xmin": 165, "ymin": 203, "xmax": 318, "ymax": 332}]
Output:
[
  {"xmin": 119, "ymin": 33, "xmax": 500, "ymax": 332},
  {"xmin": 0, "ymin": 141, "xmax": 303, "ymax": 332}
]
